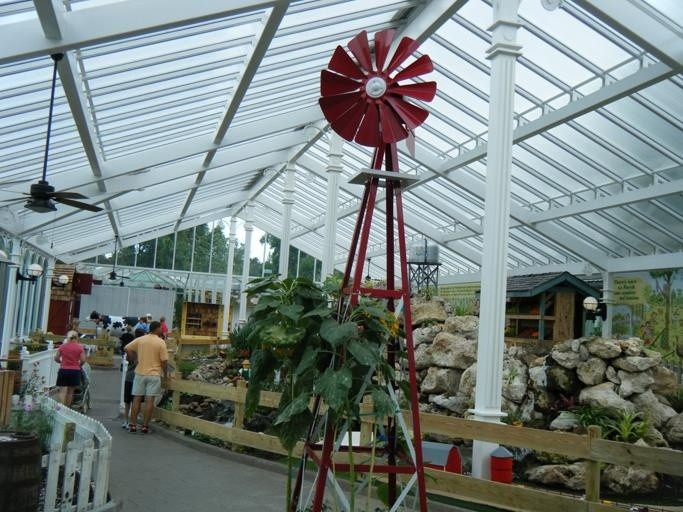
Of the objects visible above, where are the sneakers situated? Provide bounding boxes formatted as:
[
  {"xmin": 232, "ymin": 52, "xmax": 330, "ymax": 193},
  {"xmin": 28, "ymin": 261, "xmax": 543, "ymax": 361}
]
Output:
[{"xmin": 121, "ymin": 421, "xmax": 149, "ymax": 435}]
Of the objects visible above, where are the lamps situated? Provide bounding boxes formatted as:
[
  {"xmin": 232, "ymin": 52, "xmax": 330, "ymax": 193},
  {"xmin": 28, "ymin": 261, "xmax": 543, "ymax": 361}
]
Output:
[
  {"xmin": 582, "ymin": 296, "xmax": 607, "ymax": 321},
  {"xmin": 15, "ymin": 263, "xmax": 43, "ymax": 285},
  {"xmin": 50, "ymin": 274, "xmax": 69, "ymax": 290}
]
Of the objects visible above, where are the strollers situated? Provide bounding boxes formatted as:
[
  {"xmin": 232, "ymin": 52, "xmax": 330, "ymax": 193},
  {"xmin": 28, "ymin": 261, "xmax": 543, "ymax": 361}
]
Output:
[{"xmin": 70, "ymin": 361, "xmax": 92, "ymax": 414}]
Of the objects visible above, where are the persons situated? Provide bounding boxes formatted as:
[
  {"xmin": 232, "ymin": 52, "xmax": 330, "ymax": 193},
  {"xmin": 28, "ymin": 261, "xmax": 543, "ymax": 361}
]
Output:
[
  {"xmin": 122, "ymin": 328, "xmax": 148, "ymax": 431},
  {"xmin": 124, "ymin": 313, "xmax": 169, "ymax": 340},
  {"xmin": 54, "ymin": 328, "xmax": 86, "ymax": 407},
  {"xmin": 123, "ymin": 320, "xmax": 169, "ymax": 435}
]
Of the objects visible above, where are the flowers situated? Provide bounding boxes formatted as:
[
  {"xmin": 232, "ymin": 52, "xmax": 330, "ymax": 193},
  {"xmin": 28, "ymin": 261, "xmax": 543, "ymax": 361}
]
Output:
[{"xmin": 5, "ymin": 361, "xmax": 62, "ymax": 451}]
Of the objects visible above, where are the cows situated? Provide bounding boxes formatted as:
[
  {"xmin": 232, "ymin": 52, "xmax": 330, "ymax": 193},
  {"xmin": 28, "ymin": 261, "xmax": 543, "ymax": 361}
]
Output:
[{"xmin": 85, "ymin": 310, "xmax": 139, "ymax": 336}]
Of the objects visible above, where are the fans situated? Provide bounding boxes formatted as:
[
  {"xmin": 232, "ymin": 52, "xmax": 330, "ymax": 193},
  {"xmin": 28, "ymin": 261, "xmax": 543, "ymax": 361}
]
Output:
[{"xmin": 0, "ymin": 53, "xmax": 104, "ymax": 213}]
